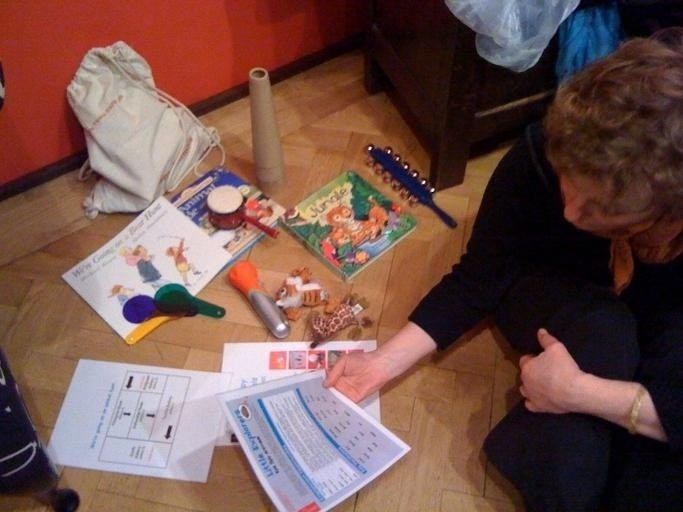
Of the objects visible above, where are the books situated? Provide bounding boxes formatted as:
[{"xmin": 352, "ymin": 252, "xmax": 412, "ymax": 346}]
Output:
[
  {"xmin": 278, "ymin": 169, "xmax": 419, "ymax": 283},
  {"xmin": 168, "ymin": 164, "xmax": 286, "ymax": 270}
]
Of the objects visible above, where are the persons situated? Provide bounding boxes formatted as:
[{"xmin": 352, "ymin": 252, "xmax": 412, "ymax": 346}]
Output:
[{"xmin": 322, "ymin": 25, "xmax": 683, "ymax": 512}]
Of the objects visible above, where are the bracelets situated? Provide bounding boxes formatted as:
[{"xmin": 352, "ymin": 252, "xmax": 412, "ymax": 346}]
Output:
[{"xmin": 629, "ymin": 386, "xmax": 647, "ymax": 434}]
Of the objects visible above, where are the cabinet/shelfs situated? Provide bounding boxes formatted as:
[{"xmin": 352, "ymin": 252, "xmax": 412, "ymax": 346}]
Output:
[{"xmin": 364, "ymin": 1, "xmax": 682, "ymax": 191}]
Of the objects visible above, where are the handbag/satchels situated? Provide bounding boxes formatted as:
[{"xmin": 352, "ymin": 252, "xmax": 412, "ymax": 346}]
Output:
[{"xmin": 65, "ymin": 38, "xmax": 229, "ymax": 223}]
